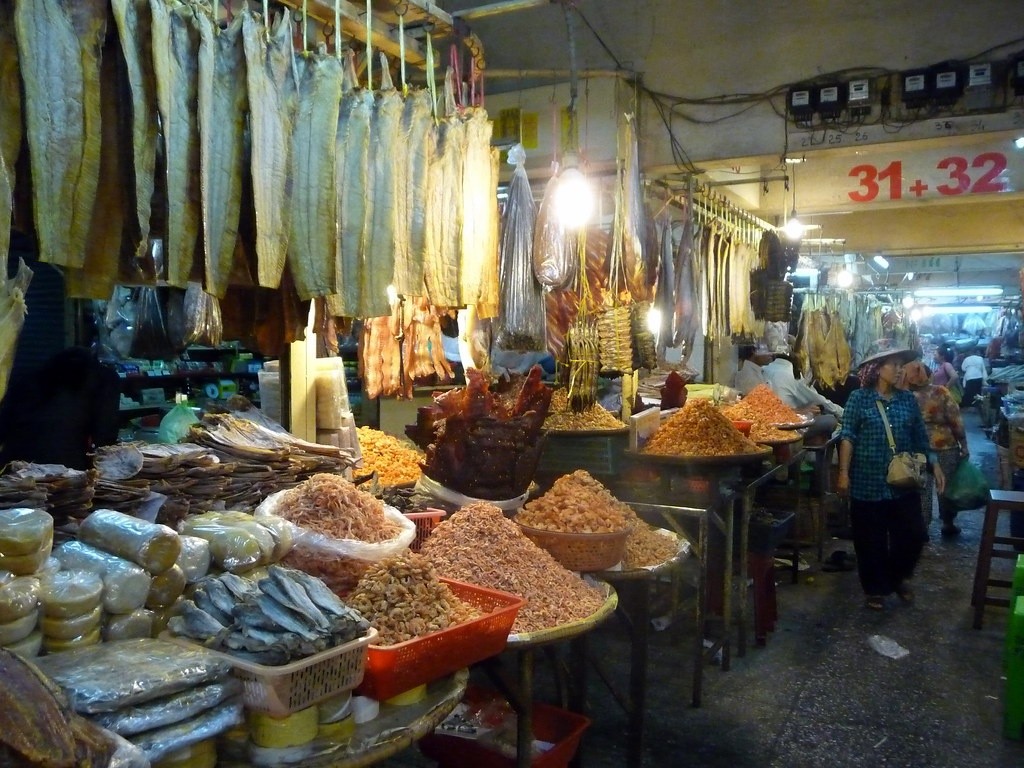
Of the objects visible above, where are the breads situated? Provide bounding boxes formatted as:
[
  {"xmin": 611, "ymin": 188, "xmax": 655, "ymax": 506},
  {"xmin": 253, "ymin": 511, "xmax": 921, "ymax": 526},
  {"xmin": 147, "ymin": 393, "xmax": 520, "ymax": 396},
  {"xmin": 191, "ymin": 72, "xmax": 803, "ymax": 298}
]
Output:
[{"xmin": 0, "ymin": 503, "xmax": 293, "ymax": 660}]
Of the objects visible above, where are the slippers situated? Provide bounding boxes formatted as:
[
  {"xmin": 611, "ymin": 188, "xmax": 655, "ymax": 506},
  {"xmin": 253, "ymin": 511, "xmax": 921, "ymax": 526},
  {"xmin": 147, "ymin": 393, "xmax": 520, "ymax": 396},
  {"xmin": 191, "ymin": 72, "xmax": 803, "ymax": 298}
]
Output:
[
  {"xmin": 867, "ymin": 596, "xmax": 880, "ymax": 610},
  {"xmin": 899, "ymin": 588, "xmax": 914, "ymax": 603},
  {"xmin": 823, "ymin": 550, "xmax": 856, "ymax": 572}
]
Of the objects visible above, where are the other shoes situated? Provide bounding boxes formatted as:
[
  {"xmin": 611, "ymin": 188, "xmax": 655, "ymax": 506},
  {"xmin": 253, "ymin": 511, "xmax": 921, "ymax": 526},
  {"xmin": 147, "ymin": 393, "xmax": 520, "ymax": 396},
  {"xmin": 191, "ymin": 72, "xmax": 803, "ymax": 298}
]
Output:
[{"xmin": 941, "ymin": 525, "xmax": 962, "ymax": 536}]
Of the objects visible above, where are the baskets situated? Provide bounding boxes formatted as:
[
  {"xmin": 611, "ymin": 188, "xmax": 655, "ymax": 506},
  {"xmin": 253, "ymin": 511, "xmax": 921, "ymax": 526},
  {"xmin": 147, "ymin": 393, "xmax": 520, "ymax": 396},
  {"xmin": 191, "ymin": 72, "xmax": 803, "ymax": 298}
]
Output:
[
  {"xmin": 158, "ymin": 627, "xmax": 379, "ymax": 720},
  {"xmin": 418, "ymin": 704, "xmax": 590, "ymax": 768},
  {"xmin": 402, "ymin": 507, "xmax": 447, "ymax": 553},
  {"xmin": 510, "ymin": 512, "xmax": 632, "ymax": 572},
  {"xmin": 366, "ymin": 576, "xmax": 528, "ymax": 701}
]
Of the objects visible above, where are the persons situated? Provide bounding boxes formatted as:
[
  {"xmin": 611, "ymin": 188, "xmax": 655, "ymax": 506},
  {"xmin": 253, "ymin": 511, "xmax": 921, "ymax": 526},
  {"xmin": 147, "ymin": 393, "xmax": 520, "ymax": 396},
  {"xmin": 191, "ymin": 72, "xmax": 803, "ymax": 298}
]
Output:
[
  {"xmin": 740, "ymin": 343, "xmax": 859, "ymax": 420},
  {"xmin": 961, "ymin": 348, "xmax": 992, "ymax": 407},
  {"xmin": 933, "ymin": 346, "xmax": 959, "ymax": 389},
  {"xmin": 836, "ymin": 338, "xmax": 945, "ymax": 608},
  {"xmin": 909, "ymin": 362, "xmax": 969, "ymax": 537}
]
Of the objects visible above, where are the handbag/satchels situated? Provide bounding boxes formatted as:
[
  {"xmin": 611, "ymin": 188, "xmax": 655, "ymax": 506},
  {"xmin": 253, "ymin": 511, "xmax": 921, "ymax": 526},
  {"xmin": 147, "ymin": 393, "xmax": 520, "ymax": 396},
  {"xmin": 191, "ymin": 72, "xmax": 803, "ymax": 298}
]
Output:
[
  {"xmin": 945, "ymin": 371, "xmax": 966, "ymax": 404},
  {"xmin": 946, "ymin": 453, "xmax": 990, "ymax": 510},
  {"xmin": 886, "ymin": 452, "xmax": 928, "ymax": 490}
]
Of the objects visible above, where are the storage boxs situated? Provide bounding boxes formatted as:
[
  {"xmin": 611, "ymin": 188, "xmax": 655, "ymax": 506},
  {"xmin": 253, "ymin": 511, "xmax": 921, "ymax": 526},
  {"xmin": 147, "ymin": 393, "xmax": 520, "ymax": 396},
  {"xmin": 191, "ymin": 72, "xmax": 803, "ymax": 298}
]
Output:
[
  {"xmin": 536, "ymin": 426, "xmax": 630, "ymax": 476},
  {"xmin": 158, "ymin": 535, "xmax": 594, "ymax": 768},
  {"xmin": 749, "ymin": 504, "xmax": 797, "ymax": 555}
]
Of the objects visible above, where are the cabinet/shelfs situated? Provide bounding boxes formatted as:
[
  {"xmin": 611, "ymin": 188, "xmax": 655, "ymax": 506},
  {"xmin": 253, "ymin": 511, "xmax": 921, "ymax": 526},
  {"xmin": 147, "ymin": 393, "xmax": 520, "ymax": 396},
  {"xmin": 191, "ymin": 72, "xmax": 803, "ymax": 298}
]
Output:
[{"xmin": 117, "ymin": 344, "xmax": 278, "ymax": 434}]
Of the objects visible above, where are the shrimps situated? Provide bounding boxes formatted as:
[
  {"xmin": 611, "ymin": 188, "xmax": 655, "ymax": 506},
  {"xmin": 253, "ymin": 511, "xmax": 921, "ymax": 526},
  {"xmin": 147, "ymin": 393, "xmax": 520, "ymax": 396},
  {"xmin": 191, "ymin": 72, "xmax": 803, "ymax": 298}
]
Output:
[
  {"xmin": 637, "ymin": 385, "xmax": 803, "ymax": 456},
  {"xmin": 341, "ymin": 470, "xmax": 677, "ymax": 641}
]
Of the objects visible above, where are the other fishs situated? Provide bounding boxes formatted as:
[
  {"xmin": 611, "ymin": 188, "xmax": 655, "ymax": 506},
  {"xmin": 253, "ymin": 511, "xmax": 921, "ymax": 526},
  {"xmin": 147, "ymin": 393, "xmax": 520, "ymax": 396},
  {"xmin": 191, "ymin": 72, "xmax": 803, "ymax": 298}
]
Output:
[{"xmin": 0, "ymin": 0, "xmax": 915, "ymax": 768}]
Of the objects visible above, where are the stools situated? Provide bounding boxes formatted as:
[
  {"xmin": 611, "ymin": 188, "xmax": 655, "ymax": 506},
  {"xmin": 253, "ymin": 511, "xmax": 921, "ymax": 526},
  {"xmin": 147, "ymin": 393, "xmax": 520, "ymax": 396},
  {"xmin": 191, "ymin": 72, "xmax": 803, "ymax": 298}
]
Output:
[
  {"xmin": 971, "ymin": 489, "xmax": 1024, "ymax": 608},
  {"xmin": 704, "ymin": 551, "xmax": 779, "ymax": 646}
]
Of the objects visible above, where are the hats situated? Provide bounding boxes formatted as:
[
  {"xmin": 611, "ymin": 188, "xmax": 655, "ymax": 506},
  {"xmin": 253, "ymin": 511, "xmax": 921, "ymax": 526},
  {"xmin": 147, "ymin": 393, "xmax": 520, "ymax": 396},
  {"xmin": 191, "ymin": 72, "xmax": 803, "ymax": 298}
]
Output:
[{"xmin": 858, "ymin": 339, "xmax": 919, "ymax": 369}]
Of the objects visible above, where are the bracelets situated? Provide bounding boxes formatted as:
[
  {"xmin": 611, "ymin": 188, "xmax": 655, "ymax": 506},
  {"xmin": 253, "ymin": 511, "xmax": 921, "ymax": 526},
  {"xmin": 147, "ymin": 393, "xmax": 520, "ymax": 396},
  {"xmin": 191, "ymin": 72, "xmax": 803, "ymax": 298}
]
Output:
[{"xmin": 840, "ymin": 468, "xmax": 849, "ymax": 470}]
